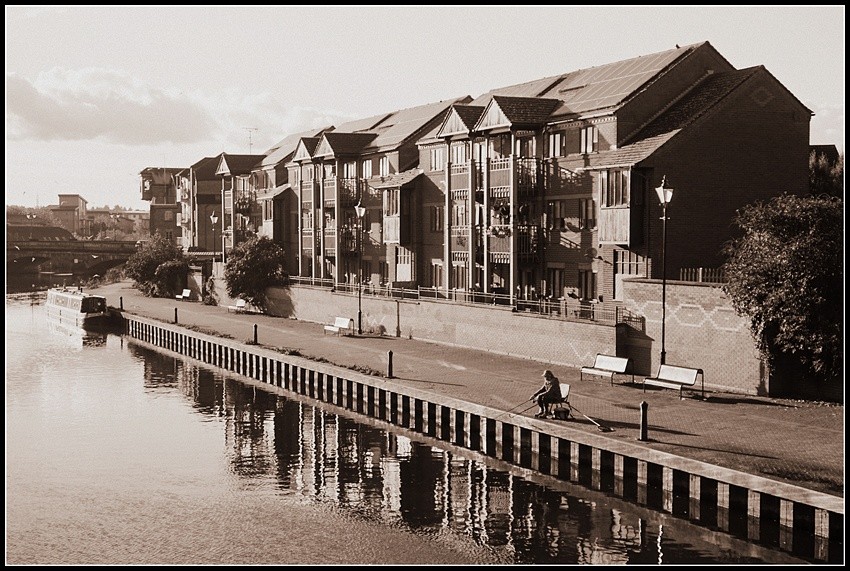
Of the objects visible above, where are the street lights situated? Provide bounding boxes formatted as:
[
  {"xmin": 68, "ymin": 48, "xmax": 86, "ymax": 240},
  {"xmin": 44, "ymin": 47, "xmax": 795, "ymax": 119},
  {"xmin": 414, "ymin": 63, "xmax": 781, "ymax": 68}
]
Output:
[
  {"xmin": 210, "ymin": 210, "xmax": 217, "ymax": 258},
  {"xmin": 657, "ymin": 176, "xmax": 675, "ymax": 361},
  {"xmin": 354, "ymin": 199, "xmax": 365, "ymax": 335}
]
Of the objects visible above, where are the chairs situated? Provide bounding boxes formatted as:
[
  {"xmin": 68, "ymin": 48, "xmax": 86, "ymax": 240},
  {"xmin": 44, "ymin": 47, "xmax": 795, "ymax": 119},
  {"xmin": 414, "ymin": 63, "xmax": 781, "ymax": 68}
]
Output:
[{"xmin": 549, "ymin": 383, "xmax": 575, "ymax": 417}]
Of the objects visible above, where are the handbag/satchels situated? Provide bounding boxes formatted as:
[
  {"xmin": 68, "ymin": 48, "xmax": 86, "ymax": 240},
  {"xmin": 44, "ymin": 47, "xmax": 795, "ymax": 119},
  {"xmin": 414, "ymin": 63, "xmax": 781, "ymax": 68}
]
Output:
[{"xmin": 554, "ymin": 407, "xmax": 571, "ymax": 420}]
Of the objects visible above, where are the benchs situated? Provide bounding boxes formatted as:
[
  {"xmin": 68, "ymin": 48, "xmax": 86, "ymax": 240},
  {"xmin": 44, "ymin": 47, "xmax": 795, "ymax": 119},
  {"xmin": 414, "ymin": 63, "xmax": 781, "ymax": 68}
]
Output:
[
  {"xmin": 580, "ymin": 353, "xmax": 634, "ymax": 387},
  {"xmin": 176, "ymin": 289, "xmax": 193, "ymax": 302},
  {"xmin": 323, "ymin": 316, "xmax": 354, "ymax": 337},
  {"xmin": 643, "ymin": 364, "xmax": 705, "ymax": 400},
  {"xmin": 227, "ymin": 300, "xmax": 251, "ymax": 314}
]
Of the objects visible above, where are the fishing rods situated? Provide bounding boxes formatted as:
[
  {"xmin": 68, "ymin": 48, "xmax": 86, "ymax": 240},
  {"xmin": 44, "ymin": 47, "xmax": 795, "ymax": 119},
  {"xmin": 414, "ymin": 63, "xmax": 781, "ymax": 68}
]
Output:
[
  {"xmin": 510, "ymin": 402, "xmax": 539, "ymax": 419},
  {"xmin": 477, "ymin": 398, "xmax": 534, "ymax": 427}
]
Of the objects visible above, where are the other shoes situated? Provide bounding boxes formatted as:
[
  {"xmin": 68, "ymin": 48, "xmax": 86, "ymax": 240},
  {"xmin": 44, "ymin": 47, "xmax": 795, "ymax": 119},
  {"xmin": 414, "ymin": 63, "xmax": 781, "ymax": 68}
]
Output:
[
  {"xmin": 534, "ymin": 408, "xmax": 544, "ymax": 416},
  {"xmin": 538, "ymin": 409, "xmax": 548, "ymax": 418}
]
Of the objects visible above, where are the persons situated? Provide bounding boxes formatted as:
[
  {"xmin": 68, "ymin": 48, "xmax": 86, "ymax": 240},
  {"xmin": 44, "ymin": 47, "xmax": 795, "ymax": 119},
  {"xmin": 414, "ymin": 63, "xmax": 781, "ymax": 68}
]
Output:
[{"xmin": 529, "ymin": 369, "xmax": 562, "ymax": 419}]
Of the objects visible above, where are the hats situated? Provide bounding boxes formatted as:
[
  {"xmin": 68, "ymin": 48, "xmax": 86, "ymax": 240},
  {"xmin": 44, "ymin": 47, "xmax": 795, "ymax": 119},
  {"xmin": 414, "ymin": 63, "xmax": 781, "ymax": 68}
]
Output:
[{"xmin": 540, "ymin": 370, "xmax": 553, "ymax": 377}]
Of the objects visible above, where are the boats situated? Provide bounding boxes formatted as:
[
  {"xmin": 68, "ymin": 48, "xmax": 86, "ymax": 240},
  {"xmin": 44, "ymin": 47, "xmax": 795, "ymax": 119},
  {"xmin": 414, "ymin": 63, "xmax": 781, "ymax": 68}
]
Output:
[{"xmin": 46, "ymin": 279, "xmax": 112, "ymax": 325}]
